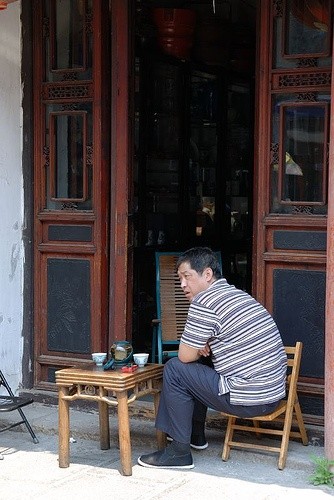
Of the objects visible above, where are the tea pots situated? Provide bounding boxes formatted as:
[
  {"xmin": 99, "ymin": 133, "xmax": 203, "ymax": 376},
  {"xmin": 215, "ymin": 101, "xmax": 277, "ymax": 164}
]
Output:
[{"xmin": 110, "ymin": 340, "xmax": 133, "ymax": 368}]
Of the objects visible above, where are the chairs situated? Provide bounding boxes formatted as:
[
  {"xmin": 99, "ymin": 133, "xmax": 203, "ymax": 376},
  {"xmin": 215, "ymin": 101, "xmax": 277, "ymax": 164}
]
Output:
[
  {"xmin": 220, "ymin": 341, "xmax": 308, "ymax": 471},
  {"xmin": 151, "ymin": 250, "xmax": 222, "ymax": 364},
  {"xmin": 0, "ymin": 370, "xmax": 39, "ymax": 461}
]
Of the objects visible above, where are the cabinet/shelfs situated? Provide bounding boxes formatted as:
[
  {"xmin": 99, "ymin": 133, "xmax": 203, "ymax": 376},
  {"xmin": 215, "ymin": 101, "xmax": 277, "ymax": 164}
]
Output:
[{"xmin": 136, "ymin": 50, "xmax": 256, "ymax": 290}]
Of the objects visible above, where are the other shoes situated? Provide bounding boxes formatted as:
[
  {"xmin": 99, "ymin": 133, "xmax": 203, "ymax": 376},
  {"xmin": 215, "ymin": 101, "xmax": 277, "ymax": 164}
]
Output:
[
  {"xmin": 167, "ymin": 434, "xmax": 208, "ymax": 450},
  {"xmin": 138, "ymin": 446, "xmax": 194, "ymax": 469}
]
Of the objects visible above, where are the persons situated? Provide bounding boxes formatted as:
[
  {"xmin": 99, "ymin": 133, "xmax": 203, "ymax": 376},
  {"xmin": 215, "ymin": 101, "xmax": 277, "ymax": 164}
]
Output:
[{"xmin": 138, "ymin": 246, "xmax": 288, "ymax": 468}]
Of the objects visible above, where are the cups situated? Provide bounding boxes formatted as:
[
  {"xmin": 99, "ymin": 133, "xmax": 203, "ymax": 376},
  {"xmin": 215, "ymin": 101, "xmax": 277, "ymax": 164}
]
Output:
[
  {"xmin": 133, "ymin": 353, "xmax": 149, "ymax": 367},
  {"xmin": 91, "ymin": 353, "xmax": 107, "ymax": 365}
]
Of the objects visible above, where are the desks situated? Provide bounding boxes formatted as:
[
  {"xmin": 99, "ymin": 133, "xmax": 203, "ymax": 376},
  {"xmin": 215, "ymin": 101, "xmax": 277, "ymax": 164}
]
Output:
[{"xmin": 53, "ymin": 361, "xmax": 168, "ymax": 476}]
